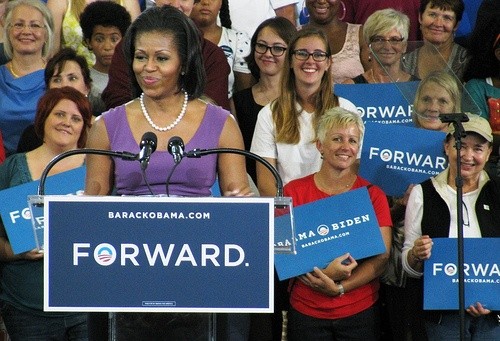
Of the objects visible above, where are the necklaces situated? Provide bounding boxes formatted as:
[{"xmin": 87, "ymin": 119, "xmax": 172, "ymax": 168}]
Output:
[
  {"xmin": 316, "ymin": 172, "xmax": 356, "ymax": 194},
  {"xmin": 370, "ymin": 67, "xmax": 401, "ymax": 83},
  {"xmin": 140, "ymin": 88, "xmax": 189, "ymax": 131},
  {"xmin": 9, "ymin": 56, "xmax": 46, "ymax": 79}
]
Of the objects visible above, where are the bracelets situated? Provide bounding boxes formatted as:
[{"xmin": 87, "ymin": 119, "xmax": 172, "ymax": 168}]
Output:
[{"xmin": 337, "ymin": 284, "xmax": 344, "ymax": 297}]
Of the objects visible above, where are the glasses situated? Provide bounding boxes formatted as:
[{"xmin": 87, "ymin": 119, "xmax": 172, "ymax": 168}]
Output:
[
  {"xmin": 254, "ymin": 42, "xmax": 288, "ymax": 57},
  {"xmin": 372, "ymin": 36, "xmax": 404, "ymax": 44},
  {"xmin": 462, "ymin": 201, "xmax": 470, "ymax": 227},
  {"xmin": 9, "ymin": 23, "xmax": 43, "ymax": 31},
  {"xmin": 292, "ymin": 49, "xmax": 330, "ymax": 62}
]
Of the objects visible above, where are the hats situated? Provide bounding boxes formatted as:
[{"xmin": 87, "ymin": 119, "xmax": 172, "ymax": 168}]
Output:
[{"xmin": 445, "ymin": 114, "xmax": 493, "ymax": 144}]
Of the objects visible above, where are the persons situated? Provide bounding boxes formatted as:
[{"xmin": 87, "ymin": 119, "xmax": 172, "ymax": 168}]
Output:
[{"xmin": 0, "ymin": 0, "xmax": 500, "ymax": 341}]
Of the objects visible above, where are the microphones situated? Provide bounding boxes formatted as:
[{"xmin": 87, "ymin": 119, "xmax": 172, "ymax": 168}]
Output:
[
  {"xmin": 38, "ymin": 131, "xmax": 158, "ymax": 195},
  {"xmin": 167, "ymin": 135, "xmax": 284, "ymax": 196}
]
